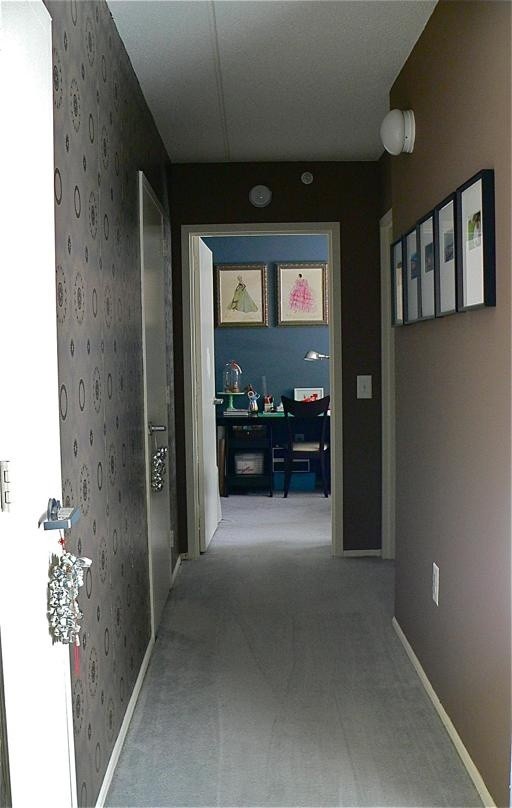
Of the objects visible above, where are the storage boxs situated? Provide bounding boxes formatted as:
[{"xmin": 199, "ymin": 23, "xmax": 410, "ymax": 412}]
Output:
[{"xmin": 271, "ymin": 469, "xmax": 317, "ymax": 492}]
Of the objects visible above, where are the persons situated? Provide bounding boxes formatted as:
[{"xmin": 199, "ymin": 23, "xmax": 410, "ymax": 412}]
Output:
[
  {"xmin": 289, "ymin": 273, "xmax": 315, "ymax": 313},
  {"xmin": 227, "ymin": 275, "xmax": 258, "ymax": 314},
  {"xmin": 471, "ymin": 213, "xmax": 479, "ymax": 249}
]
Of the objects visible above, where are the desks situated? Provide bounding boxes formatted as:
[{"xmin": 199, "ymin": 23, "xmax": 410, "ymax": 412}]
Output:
[{"xmin": 215, "ymin": 407, "xmax": 334, "ymax": 497}]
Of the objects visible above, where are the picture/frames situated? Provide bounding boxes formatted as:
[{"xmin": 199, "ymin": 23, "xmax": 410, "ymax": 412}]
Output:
[
  {"xmin": 272, "ymin": 259, "xmax": 327, "ymax": 330},
  {"xmin": 211, "ymin": 258, "xmax": 269, "ymax": 329},
  {"xmin": 385, "ymin": 166, "xmax": 499, "ymax": 331},
  {"xmin": 293, "ymin": 387, "xmax": 325, "ymax": 403}
]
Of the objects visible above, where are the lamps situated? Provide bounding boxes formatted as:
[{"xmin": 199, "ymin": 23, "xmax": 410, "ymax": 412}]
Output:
[
  {"xmin": 303, "ymin": 349, "xmax": 330, "ymax": 362},
  {"xmin": 370, "ymin": 104, "xmax": 420, "ymax": 159},
  {"xmin": 247, "ymin": 184, "xmax": 274, "ymax": 208}
]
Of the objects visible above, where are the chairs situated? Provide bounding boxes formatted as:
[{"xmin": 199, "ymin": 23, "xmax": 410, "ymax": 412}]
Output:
[{"xmin": 280, "ymin": 393, "xmax": 331, "ymax": 498}]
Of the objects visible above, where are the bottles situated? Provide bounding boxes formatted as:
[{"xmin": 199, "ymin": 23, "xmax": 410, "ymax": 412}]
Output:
[
  {"xmin": 250, "ymin": 396, "xmax": 259, "ymax": 414},
  {"xmin": 222, "ymin": 361, "xmax": 240, "ymax": 393}
]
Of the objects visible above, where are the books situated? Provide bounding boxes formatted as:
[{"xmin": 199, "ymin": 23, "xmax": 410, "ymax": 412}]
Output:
[{"xmin": 222, "ymin": 410, "xmax": 248, "ymax": 416}]
